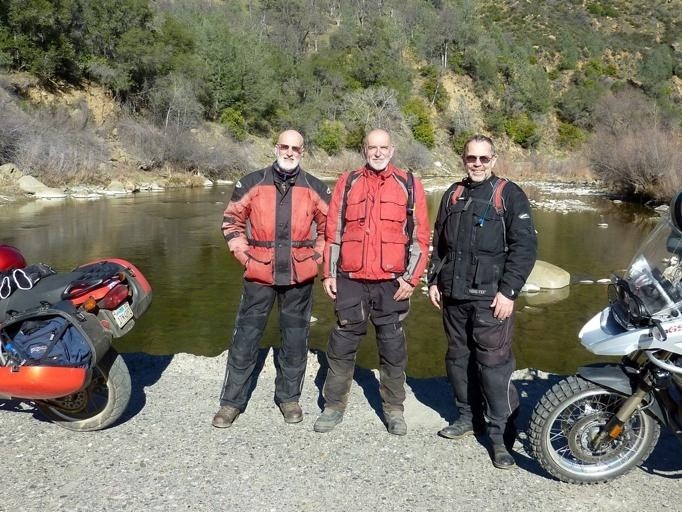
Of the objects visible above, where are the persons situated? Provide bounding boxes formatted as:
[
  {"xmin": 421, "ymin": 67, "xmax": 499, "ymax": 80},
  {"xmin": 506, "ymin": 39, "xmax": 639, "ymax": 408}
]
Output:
[
  {"xmin": 315, "ymin": 129, "xmax": 429, "ymax": 437},
  {"xmin": 210, "ymin": 129, "xmax": 334, "ymax": 428},
  {"xmin": 427, "ymin": 136, "xmax": 538, "ymax": 468}
]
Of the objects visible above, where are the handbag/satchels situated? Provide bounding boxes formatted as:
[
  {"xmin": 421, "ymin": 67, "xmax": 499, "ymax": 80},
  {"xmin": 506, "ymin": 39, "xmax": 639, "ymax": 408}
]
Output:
[{"xmin": 13, "ymin": 318, "xmax": 92, "ymax": 366}]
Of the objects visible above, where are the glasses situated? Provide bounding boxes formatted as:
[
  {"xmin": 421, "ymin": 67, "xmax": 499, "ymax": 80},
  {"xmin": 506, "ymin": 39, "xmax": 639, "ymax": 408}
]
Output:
[
  {"xmin": 464, "ymin": 154, "xmax": 490, "ymax": 164},
  {"xmin": 277, "ymin": 143, "xmax": 302, "ymax": 154}
]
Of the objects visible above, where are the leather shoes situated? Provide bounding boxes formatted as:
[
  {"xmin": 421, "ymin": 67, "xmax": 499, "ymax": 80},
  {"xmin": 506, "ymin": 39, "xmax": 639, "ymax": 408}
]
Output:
[
  {"xmin": 488, "ymin": 433, "xmax": 515, "ymax": 469},
  {"xmin": 439, "ymin": 414, "xmax": 487, "ymax": 439}
]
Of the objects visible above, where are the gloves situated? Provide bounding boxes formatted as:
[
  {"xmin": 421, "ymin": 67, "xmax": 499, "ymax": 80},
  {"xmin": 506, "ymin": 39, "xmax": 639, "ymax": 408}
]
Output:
[{"xmin": 0, "ymin": 262, "xmax": 56, "ymax": 300}]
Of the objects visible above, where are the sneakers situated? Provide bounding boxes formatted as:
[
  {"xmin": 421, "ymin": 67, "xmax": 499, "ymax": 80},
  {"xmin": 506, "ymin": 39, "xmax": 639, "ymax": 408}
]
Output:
[
  {"xmin": 384, "ymin": 411, "xmax": 407, "ymax": 436},
  {"xmin": 212, "ymin": 406, "xmax": 240, "ymax": 428},
  {"xmin": 280, "ymin": 402, "xmax": 303, "ymax": 423},
  {"xmin": 314, "ymin": 408, "xmax": 343, "ymax": 433}
]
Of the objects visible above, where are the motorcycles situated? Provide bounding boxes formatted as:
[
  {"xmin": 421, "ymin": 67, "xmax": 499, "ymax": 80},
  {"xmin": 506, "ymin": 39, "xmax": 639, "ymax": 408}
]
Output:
[
  {"xmin": 527, "ymin": 191, "xmax": 681, "ymax": 486},
  {"xmin": 0, "ymin": 243, "xmax": 155, "ymax": 432}
]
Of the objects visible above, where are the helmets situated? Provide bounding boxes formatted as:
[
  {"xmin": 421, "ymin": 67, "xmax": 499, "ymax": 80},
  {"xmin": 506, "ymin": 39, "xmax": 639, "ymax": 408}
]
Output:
[{"xmin": 0, "ymin": 244, "xmax": 26, "ymax": 275}]
